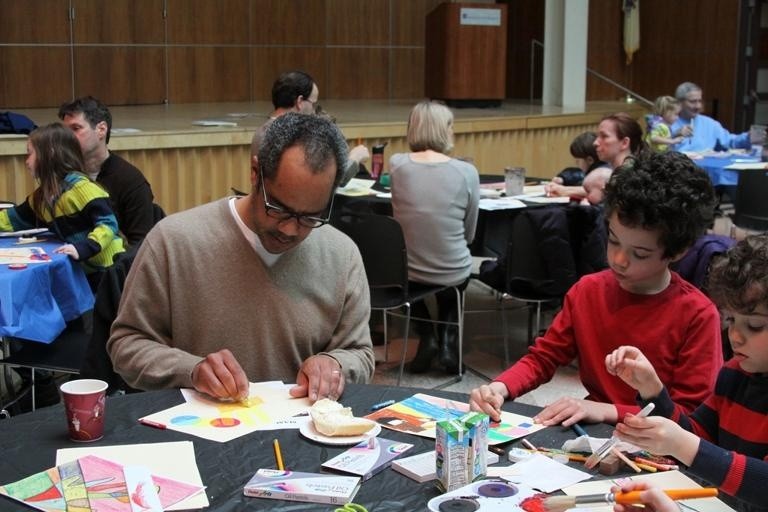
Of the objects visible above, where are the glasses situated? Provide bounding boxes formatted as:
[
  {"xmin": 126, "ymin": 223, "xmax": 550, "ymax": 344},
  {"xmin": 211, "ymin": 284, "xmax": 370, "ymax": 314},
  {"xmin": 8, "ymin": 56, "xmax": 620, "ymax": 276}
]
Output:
[
  {"xmin": 304, "ymin": 98, "xmax": 318, "ymax": 108},
  {"xmin": 259, "ymin": 165, "xmax": 335, "ymax": 228}
]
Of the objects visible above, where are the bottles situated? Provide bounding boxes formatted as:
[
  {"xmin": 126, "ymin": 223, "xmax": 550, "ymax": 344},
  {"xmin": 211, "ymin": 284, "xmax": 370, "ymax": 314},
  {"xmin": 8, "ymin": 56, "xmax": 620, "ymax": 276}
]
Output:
[{"xmin": 372, "ymin": 146, "xmax": 385, "ymax": 178}]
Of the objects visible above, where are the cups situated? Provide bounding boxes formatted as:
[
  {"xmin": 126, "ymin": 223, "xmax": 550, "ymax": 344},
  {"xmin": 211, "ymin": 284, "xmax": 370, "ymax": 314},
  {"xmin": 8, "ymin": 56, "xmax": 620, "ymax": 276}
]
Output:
[
  {"xmin": 61, "ymin": 380, "xmax": 107, "ymax": 442},
  {"xmin": 504, "ymin": 167, "xmax": 526, "ymax": 196}
]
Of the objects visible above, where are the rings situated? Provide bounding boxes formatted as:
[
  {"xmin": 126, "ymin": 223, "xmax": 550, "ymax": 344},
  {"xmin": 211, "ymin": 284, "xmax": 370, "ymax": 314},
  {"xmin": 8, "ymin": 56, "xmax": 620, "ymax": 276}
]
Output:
[{"xmin": 332, "ymin": 370, "xmax": 341, "ymax": 374}]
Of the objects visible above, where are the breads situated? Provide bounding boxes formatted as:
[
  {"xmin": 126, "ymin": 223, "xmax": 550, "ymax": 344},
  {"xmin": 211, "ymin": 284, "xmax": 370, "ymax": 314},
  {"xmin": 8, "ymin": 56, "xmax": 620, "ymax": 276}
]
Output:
[{"xmin": 309, "ymin": 398, "xmax": 376, "ymax": 436}]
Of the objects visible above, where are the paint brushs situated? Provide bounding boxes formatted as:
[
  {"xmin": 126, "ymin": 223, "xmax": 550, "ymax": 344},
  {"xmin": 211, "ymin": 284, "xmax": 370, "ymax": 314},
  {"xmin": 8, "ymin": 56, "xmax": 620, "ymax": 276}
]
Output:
[
  {"xmin": 584, "ymin": 402, "xmax": 656, "ymax": 469},
  {"xmin": 519, "ymin": 487, "xmax": 719, "ymax": 512}
]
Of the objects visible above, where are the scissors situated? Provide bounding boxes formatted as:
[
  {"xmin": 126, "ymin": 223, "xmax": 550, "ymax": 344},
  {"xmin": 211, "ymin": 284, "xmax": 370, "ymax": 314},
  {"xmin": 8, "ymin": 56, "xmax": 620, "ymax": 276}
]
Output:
[{"xmin": 334, "ymin": 503, "xmax": 369, "ymax": 512}]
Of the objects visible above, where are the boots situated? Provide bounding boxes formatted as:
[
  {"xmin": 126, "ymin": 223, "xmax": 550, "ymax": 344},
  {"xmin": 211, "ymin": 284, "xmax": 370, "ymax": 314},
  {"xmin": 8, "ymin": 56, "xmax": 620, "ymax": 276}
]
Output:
[{"xmin": 403, "ymin": 318, "xmax": 466, "ymax": 376}]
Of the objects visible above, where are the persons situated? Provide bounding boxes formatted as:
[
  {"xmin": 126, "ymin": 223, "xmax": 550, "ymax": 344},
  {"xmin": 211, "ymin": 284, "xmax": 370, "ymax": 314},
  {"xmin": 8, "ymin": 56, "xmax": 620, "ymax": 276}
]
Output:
[
  {"xmin": 650, "ymin": 80, "xmax": 767, "ymax": 215},
  {"xmin": 603, "ymin": 230, "xmax": 768, "ymax": 511},
  {"xmin": 57, "ymin": 95, "xmax": 155, "ymax": 249},
  {"xmin": 550, "ymin": 130, "xmax": 611, "ymax": 185},
  {"xmin": 544, "ymin": 111, "xmax": 646, "ymax": 209},
  {"xmin": 387, "ymin": 100, "xmax": 481, "ymax": 376},
  {"xmin": 102, "ymin": 110, "xmax": 376, "ymax": 407},
  {"xmin": 605, "ymin": 475, "xmax": 683, "ymax": 512},
  {"xmin": 248, "ymin": 70, "xmax": 369, "ymax": 208},
  {"xmin": 466, "ymin": 149, "xmax": 724, "ymax": 429},
  {"xmin": 0, "ymin": 122, "xmax": 135, "ymax": 297},
  {"xmin": 641, "ymin": 94, "xmax": 694, "ymax": 154},
  {"xmin": 578, "ymin": 167, "xmax": 613, "ymax": 209}
]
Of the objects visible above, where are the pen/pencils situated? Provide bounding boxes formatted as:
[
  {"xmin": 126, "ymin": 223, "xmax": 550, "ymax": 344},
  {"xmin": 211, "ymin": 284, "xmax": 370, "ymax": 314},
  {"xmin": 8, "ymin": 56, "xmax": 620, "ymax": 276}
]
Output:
[
  {"xmin": 274, "ymin": 438, "xmax": 284, "ymax": 470},
  {"xmin": 688, "ymin": 118, "xmax": 694, "ymax": 144},
  {"xmin": 140, "ymin": 418, "xmax": 166, "ymax": 429},
  {"xmin": 370, "ymin": 400, "xmax": 395, "ymax": 411},
  {"xmin": 521, "ymin": 423, "xmax": 679, "ymax": 473}
]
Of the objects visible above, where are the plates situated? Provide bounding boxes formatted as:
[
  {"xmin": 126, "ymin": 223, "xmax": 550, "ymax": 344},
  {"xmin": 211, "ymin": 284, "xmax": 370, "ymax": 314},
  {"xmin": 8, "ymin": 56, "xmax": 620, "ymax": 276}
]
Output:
[{"xmin": 298, "ymin": 418, "xmax": 382, "ymax": 445}]
